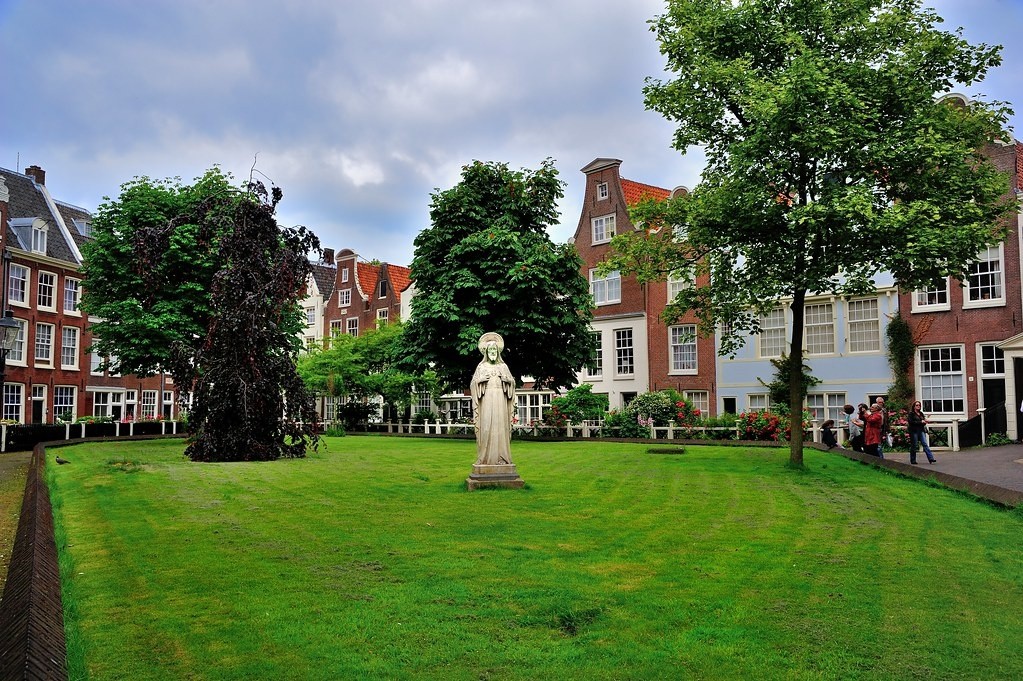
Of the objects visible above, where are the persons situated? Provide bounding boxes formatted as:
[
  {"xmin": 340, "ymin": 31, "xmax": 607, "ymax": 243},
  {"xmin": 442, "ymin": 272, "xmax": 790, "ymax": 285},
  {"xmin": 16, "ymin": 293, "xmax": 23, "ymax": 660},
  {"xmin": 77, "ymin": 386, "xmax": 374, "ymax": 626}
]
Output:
[
  {"xmin": 820, "ymin": 420, "xmax": 844, "ymax": 449},
  {"xmin": 907, "ymin": 401, "xmax": 938, "ymax": 464},
  {"xmin": 841, "ymin": 397, "xmax": 886, "ymax": 458},
  {"xmin": 470, "ymin": 332, "xmax": 518, "ymax": 464}
]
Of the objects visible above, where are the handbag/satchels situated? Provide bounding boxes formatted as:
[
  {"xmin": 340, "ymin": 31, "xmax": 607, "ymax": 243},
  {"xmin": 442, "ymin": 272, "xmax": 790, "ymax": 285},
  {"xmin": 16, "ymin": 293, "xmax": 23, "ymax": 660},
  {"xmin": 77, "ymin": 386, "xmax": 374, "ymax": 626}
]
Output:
[
  {"xmin": 923, "ymin": 426, "xmax": 928, "ymax": 433},
  {"xmin": 886, "ymin": 432, "xmax": 892, "ymax": 447}
]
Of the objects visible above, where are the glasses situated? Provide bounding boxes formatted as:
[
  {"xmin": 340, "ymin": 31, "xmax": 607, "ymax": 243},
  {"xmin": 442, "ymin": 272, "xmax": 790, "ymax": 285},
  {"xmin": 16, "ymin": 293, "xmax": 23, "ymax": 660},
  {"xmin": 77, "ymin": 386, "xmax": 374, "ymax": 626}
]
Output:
[{"xmin": 915, "ymin": 405, "xmax": 920, "ymax": 406}]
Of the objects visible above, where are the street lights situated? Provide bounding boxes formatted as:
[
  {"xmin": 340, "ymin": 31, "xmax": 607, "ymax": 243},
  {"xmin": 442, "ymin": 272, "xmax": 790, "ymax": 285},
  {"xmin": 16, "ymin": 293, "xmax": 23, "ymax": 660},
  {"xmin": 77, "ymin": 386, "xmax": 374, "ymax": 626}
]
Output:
[{"xmin": 0, "ymin": 305, "xmax": 23, "ymax": 450}]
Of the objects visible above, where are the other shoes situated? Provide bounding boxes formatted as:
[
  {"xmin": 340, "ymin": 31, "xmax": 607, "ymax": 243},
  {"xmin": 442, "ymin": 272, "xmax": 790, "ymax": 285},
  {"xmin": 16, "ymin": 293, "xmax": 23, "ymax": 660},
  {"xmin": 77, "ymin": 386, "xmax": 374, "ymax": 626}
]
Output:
[
  {"xmin": 911, "ymin": 462, "xmax": 918, "ymax": 464},
  {"xmin": 929, "ymin": 459, "xmax": 936, "ymax": 464}
]
